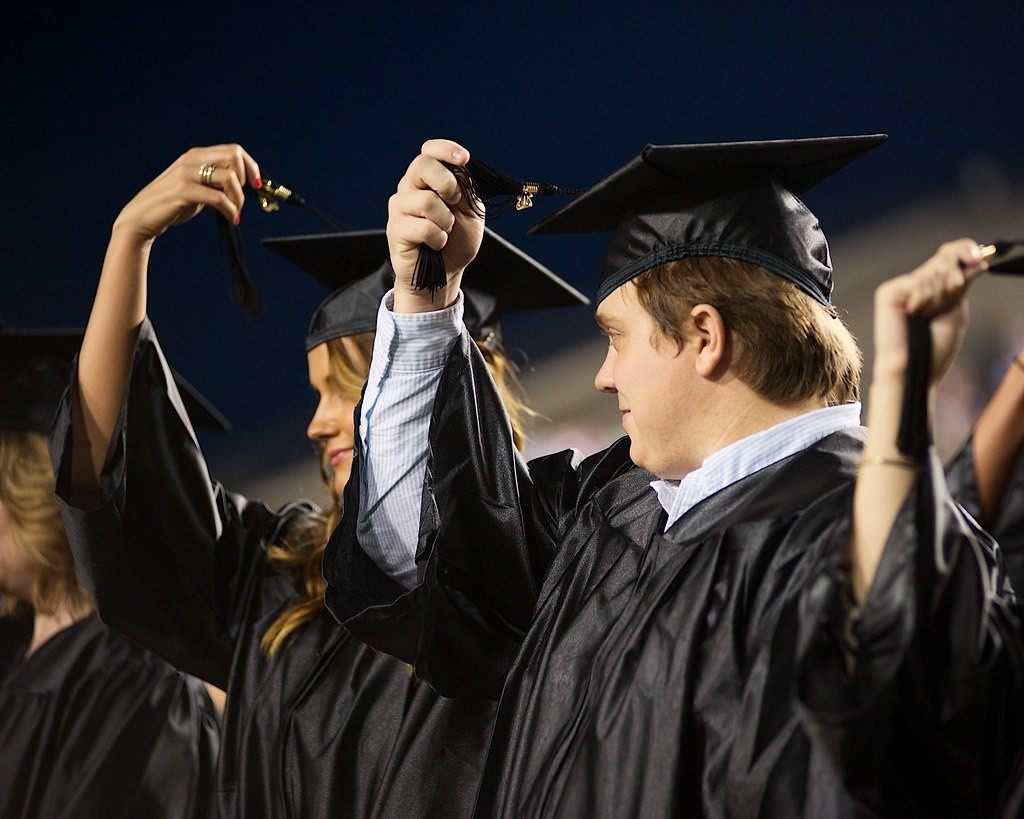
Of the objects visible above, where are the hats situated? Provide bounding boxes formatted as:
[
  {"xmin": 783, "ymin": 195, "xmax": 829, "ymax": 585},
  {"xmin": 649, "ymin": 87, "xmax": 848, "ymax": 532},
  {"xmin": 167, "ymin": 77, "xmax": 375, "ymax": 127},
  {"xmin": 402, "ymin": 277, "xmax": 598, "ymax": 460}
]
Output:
[
  {"xmin": 218, "ymin": 176, "xmax": 591, "ymax": 356},
  {"xmin": 0, "ymin": 326, "xmax": 230, "ymax": 440},
  {"xmin": 409, "ymin": 132, "xmax": 890, "ymax": 316}
]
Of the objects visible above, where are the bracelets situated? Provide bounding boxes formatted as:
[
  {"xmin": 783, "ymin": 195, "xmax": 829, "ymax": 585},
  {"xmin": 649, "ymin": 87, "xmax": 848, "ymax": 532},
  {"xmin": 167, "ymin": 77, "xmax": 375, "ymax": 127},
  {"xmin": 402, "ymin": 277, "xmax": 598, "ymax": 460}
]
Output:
[
  {"xmin": 1012, "ymin": 355, "xmax": 1024, "ymax": 371},
  {"xmin": 856, "ymin": 458, "xmax": 918, "ymax": 471}
]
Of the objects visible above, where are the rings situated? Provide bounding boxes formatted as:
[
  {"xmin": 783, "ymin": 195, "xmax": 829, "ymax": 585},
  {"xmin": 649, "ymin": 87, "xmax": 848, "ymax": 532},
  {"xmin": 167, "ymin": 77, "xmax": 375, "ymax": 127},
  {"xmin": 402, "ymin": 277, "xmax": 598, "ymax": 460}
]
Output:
[{"xmin": 198, "ymin": 163, "xmax": 219, "ymax": 186}]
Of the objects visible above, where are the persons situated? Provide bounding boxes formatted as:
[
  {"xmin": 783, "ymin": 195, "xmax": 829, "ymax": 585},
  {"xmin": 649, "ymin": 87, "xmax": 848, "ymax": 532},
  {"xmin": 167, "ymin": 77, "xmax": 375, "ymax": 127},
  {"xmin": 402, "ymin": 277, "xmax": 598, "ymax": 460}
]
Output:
[{"xmin": 0, "ymin": 139, "xmax": 1024, "ymax": 819}]
